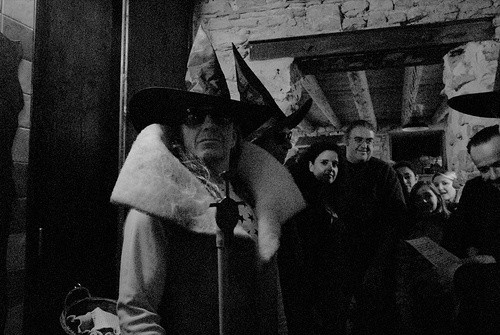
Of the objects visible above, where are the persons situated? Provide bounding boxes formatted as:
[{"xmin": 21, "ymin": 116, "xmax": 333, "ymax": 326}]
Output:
[
  {"xmin": 110, "ymin": 23, "xmax": 313, "ymax": 335},
  {"xmin": 431, "ymin": 172, "xmax": 454, "ymax": 207},
  {"xmin": 443, "ymin": 125, "xmax": 500, "ymax": 335},
  {"xmin": 394, "ymin": 179, "xmax": 451, "ymax": 335},
  {"xmin": 322, "ymin": 119, "xmax": 407, "ymax": 335},
  {"xmin": 393, "ymin": 162, "xmax": 419, "ymax": 202},
  {"xmin": 276, "ymin": 144, "xmax": 345, "ymax": 335}
]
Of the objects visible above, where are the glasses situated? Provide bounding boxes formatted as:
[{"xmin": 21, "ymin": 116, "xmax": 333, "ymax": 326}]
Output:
[
  {"xmin": 178, "ymin": 107, "xmax": 233, "ymax": 127},
  {"xmin": 274, "ymin": 132, "xmax": 292, "ymax": 141}
]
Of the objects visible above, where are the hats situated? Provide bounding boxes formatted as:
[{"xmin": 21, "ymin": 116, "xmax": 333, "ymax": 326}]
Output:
[
  {"xmin": 225, "ymin": 38, "xmax": 313, "ymax": 143},
  {"xmin": 128, "ymin": 25, "xmax": 270, "ymax": 140},
  {"xmin": 446, "ymin": 48, "xmax": 500, "ymax": 118}
]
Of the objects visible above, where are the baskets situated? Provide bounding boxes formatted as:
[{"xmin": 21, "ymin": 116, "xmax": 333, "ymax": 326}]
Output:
[{"xmin": 60, "ymin": 286, "xmax": 117, "ymax": 335}]
